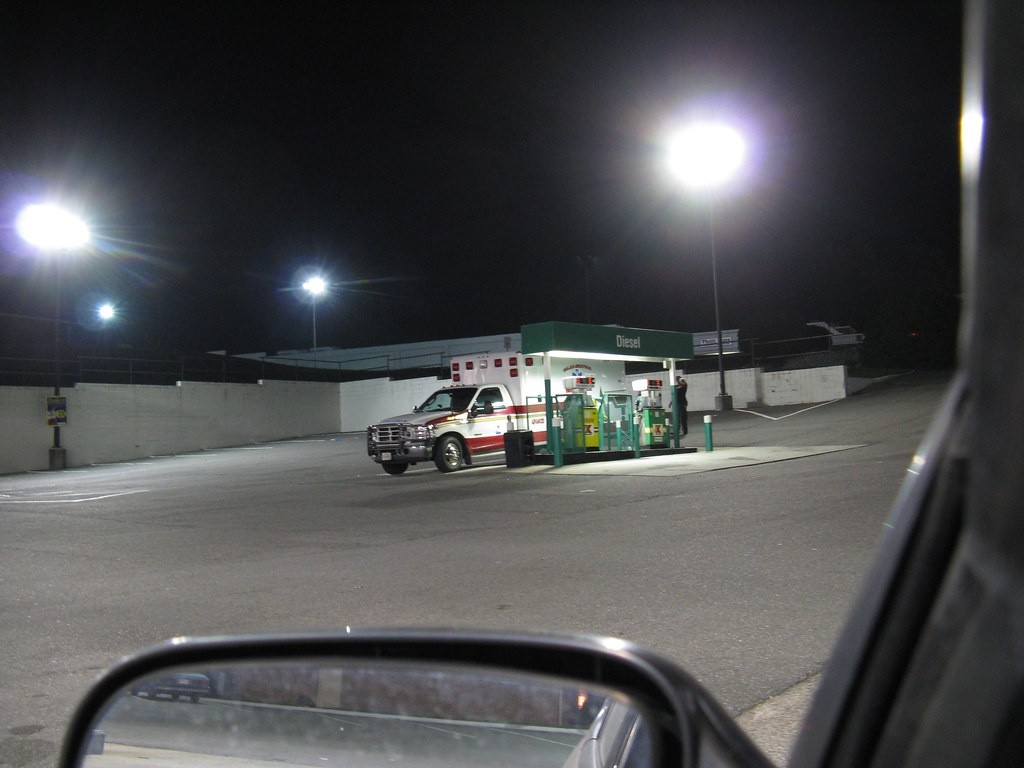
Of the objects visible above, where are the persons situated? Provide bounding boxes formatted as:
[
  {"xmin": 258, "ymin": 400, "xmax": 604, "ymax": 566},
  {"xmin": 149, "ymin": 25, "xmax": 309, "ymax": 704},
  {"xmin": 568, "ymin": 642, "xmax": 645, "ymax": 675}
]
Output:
[{"xmin": 669, "ymin": 376, "xmax": 688, "ymax": 435}]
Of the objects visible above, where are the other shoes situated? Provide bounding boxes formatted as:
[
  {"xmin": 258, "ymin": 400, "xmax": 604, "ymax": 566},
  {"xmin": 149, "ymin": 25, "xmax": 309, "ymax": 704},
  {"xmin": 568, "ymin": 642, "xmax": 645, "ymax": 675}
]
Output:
[
  {"xmin": 670, "ymin": 431, "xmax": 674, "ymax": 434},
  {"xmin": 680, "ymin": 431, "xmax": 688, "ymax": 436}
]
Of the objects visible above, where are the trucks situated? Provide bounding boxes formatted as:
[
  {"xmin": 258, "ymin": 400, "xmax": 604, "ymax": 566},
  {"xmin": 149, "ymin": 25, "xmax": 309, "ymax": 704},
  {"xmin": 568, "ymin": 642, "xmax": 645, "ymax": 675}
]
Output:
[{"xmin": 366, "ymin": 348, "xmax": 628, "ymax": 477}]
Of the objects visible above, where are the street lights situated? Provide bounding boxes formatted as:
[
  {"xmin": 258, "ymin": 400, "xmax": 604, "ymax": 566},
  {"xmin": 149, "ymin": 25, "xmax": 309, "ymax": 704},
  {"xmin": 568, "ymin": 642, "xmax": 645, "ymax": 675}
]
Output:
[
  {"xmin": 13, "ymin": 205, "xmax": 91, "ymax": 467},
  {"xmin": 677, "ymin": 123, "xmax": 746, "ymax": 413}
]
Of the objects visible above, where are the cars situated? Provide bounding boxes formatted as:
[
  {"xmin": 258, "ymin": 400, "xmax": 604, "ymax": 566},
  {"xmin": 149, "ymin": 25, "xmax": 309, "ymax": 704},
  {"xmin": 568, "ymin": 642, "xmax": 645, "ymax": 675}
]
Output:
[{"xmin": 56, "ymin": 370, "xmax": 1024, "ymax": 768}]
